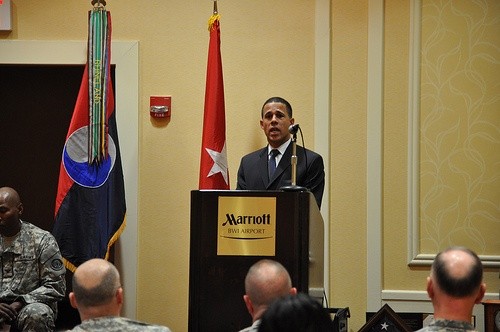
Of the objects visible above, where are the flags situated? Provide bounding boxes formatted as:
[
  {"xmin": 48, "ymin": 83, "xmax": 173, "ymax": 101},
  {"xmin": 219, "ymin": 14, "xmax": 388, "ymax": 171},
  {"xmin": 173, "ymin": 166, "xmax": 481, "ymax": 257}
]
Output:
[
  {"xmin": 52, "ymin": 24, "xmax": 128, "ymax": 279},
  {"xmin": 198, "ymin": 24, "xmax": 231, "ymax": 190}
]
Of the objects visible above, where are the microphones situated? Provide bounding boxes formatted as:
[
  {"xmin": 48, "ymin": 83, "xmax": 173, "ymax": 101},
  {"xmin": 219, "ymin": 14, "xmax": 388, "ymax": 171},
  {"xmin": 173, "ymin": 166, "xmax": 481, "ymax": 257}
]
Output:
[{"xmin": 288, "ymin": 124, "xmax": 299, "ymax": 134}]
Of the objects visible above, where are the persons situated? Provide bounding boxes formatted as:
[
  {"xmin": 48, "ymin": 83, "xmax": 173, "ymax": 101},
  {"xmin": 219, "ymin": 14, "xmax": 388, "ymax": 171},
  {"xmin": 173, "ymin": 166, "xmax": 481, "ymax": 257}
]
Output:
[
  {"xmin": 234, "ymin": 97, "xmax": 325, "ymax": 213},
  {"xmin": 236, "ymin": 259, "xmax": 335, "ymax": 332},
  {"xmin": 0, "ymin": 187, "xmax": 66, "ymax": 332},
  {"xmin": 412, "ymin": 246, "xmax": 486, "ymax": 332},
  {"xmin": 62, "ymin": 258, "xmax": 171, "ymax": 332}
]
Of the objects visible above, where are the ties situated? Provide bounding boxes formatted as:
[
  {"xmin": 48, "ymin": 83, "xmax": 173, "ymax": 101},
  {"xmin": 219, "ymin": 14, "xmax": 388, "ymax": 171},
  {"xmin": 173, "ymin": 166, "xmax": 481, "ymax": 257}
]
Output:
[{"xmin": 268, "ymin": 150, "xmax": 280, "ymax": 178}]
